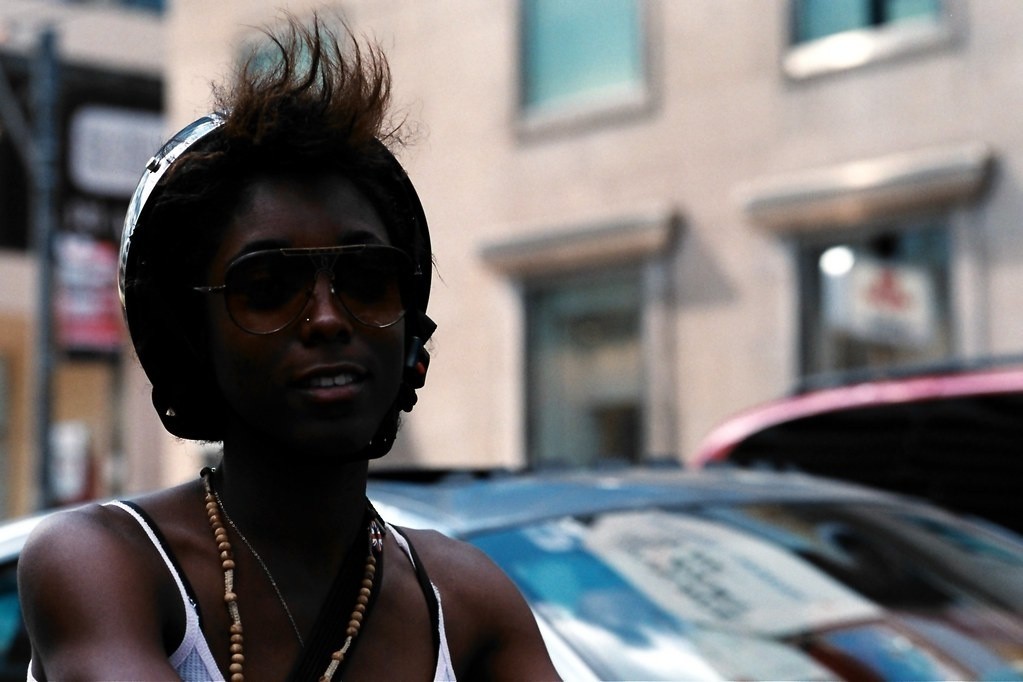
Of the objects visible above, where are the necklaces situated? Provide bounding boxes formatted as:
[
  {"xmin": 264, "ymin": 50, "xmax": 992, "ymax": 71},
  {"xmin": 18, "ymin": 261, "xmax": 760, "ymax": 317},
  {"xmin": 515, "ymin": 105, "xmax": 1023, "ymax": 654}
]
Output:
[
  {"xmin": 199, "ymin": 458, "xmax": 385, "ymax": 682},
  {"xmin": 214, "ymin": 481, "xmax": 331, "ymax": 658}
]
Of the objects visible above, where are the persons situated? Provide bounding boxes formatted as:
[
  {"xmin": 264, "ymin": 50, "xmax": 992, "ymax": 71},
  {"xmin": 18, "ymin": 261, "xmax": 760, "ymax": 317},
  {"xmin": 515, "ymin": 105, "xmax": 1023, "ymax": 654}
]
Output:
[{"xmin": 19, "ymin": 11, "xmax": 560, "ymax": 682}]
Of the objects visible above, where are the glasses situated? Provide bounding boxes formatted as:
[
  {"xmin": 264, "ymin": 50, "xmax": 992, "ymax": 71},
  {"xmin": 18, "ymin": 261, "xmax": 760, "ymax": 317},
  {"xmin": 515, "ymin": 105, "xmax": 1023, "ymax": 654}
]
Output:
[{"xmin": 180, "ymin": 243, "xmax": 423, "ymax": 336}]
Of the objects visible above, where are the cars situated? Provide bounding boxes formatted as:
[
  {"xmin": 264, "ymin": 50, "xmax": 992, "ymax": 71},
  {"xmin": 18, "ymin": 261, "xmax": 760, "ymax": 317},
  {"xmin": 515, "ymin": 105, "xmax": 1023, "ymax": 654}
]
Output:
[
  {"xmin": 0, "ymin": 456, "xmax": 1023, "ymax": 682},
  {"xmin": 685, "ymin": 360, "xmax": 1023, "ymax": 547}
]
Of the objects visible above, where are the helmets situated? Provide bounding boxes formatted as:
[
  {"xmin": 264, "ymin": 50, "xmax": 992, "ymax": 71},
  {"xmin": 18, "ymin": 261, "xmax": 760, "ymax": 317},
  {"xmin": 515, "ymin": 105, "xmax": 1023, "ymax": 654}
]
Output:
[{"xmin": 117, "ymin": 108, "xmax": 433, "ymax": 430}]
